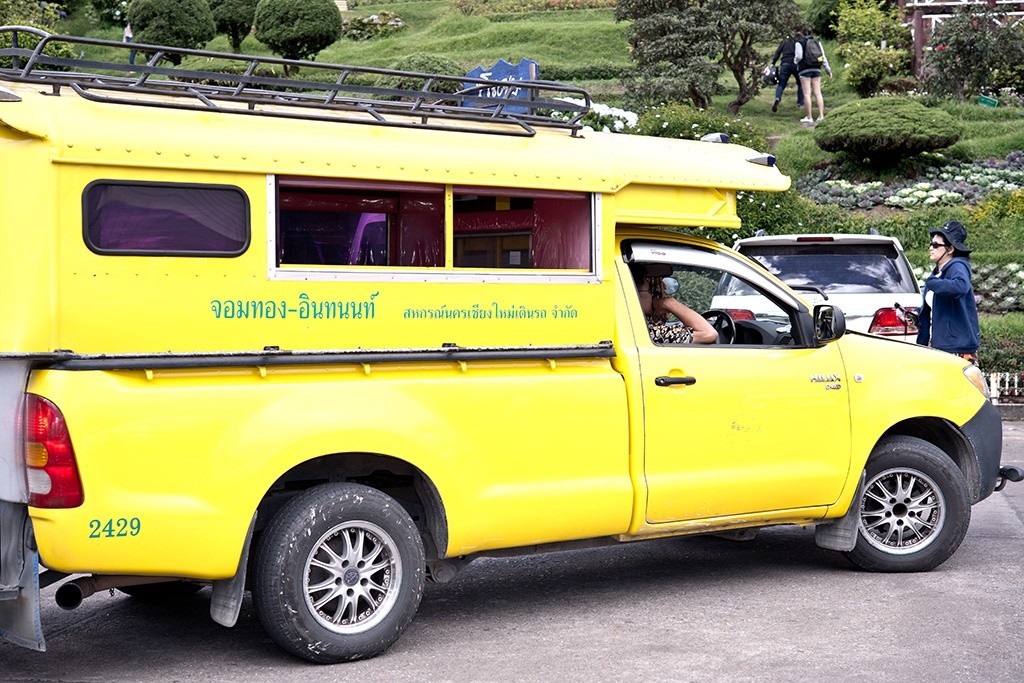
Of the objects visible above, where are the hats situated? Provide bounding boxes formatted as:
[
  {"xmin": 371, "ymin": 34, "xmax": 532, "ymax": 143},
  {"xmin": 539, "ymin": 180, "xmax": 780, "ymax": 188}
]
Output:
[{"xmin": 930, "ymin": 221, "xmax": 972, "ymax": 256}]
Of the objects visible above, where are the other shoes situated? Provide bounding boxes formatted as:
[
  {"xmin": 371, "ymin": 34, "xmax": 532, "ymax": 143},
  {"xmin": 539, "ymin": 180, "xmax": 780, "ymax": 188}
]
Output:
[{"xmin": 772, "ymin": 100, "xmax": 779, "ymax": 112}]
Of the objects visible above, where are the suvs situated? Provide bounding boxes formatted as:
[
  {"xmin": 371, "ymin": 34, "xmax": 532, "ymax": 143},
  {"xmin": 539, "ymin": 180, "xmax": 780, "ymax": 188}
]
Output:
[{"xmin": 707, "ymin": 227, "xmax": 927, "ymax": 345}]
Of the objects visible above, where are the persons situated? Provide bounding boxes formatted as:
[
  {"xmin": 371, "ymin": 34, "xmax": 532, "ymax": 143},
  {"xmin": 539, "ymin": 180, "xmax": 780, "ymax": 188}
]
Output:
[
  {"xmin": 794, "ymin": 24, "xmax": 832, "ymax": 123},
  {"xmin": 121, "ymin": 14, "xmax": 134, "ymax": 43},
  {"xmin": 769, "ymin": 37, "xmax": 804, "ymax": 112},
  {"xmin": 906, "ymin": 221, "xmax": 979, "ymax": 368},
  {"xmin": 631, "ymin": 261, "xmax": 718, "ymax": 345}
]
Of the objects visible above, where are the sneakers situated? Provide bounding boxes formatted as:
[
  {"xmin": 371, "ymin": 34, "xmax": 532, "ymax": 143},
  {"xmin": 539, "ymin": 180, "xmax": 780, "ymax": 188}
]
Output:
[
  {"xmin": 800, "ymin": 116, "xmax": 813, "ymax": 123},
  {"xmin": 815, "ymin": 117, "xmax": 822, "ymax": 122}
]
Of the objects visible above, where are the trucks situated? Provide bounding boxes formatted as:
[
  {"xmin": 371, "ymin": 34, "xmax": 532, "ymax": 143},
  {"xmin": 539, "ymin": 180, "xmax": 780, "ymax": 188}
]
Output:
[{"xmin": 1, "ymin": 22, "xmax": 1021, "ymax": 664}]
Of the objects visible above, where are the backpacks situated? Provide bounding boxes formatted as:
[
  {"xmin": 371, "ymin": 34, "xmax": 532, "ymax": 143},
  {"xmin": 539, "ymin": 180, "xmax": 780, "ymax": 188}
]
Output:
[{"xmin": 804, "ymin": 37, "xmax": 824, "ymax": 67}]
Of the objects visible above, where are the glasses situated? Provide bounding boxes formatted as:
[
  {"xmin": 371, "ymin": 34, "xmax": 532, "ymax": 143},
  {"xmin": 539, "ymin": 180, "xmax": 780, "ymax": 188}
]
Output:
[
  {"xmin": 929, "ymin": 240, "xmax": 946, "ymax": 248},
  {"xmin": 641, "ymin": 288, "xmax": 653, "ymax": 295}
]
